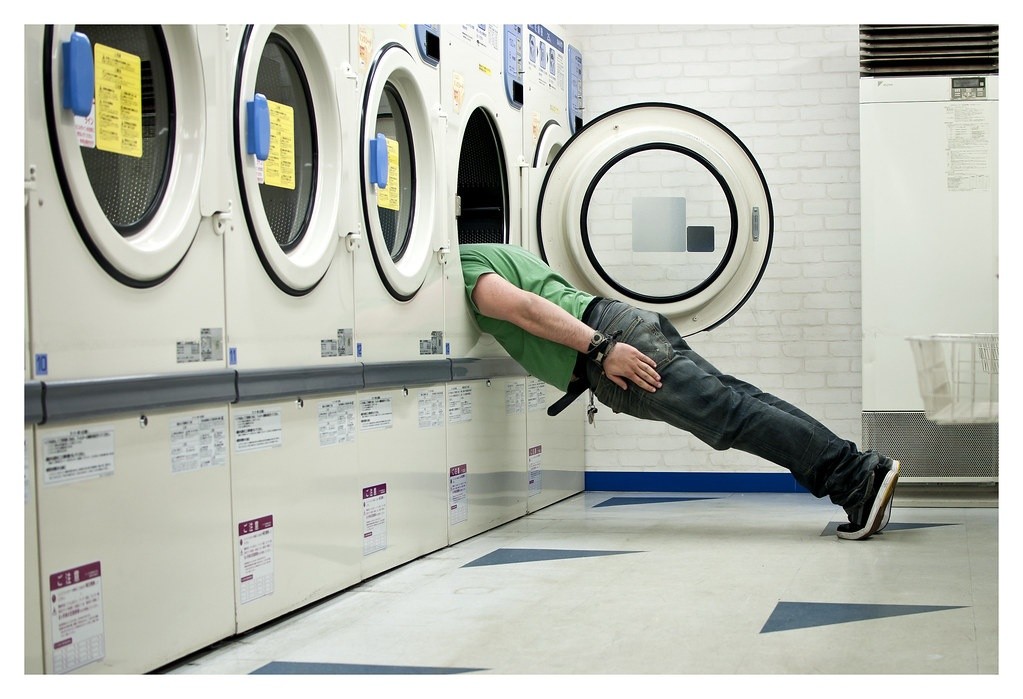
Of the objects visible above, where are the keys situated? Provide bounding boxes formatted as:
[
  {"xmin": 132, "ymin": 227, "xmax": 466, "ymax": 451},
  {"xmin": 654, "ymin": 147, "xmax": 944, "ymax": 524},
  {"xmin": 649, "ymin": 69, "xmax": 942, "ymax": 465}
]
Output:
[{"xmin": 588, "ymin": 408, "xmax": 597, "ymax": 424}]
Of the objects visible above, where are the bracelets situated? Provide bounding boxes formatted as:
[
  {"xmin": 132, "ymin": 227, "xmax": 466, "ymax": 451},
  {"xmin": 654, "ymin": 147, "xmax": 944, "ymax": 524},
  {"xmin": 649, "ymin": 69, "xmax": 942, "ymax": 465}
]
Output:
[{"xmin": 587, "ymin": 330, "xmax": 622, "ymax": 367}]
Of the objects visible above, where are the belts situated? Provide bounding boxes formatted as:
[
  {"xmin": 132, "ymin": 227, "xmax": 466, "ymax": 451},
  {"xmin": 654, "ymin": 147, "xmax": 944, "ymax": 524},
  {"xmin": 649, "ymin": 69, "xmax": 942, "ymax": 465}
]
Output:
[{"xmin": 547, "ymin": 297, "xmax": 618, "ymax": 416}]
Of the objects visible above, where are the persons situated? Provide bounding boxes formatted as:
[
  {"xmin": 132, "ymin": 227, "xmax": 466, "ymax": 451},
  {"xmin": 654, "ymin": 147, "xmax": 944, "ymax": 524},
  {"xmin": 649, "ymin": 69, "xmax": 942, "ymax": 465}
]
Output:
[{"xmin": 459, "ymin": 243, "xmax": 901, "ymax": 540}]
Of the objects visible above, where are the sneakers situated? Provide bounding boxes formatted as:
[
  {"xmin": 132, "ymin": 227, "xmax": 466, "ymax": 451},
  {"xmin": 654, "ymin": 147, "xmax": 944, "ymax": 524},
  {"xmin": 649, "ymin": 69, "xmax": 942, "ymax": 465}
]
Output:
[
  {"xmin": 883, "ymin": 456, "xmax": 901, "ymax": 472},
  {"xmin": 837, "ymin": 469, "xmax": 899, "ymax": 540}
]
